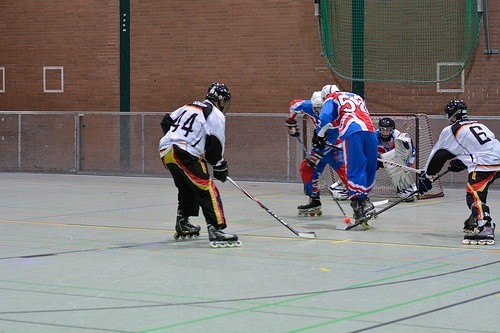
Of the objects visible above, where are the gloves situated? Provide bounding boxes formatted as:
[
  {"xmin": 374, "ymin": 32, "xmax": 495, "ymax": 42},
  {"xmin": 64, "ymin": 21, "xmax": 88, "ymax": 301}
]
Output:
[
  {"xmin": 285, "ymin": 120, "xmax": 299, "ymax": 136},
  {"xmin": 448, "ymin": 159, "xmax": 467, "ymax": 172},
  {"xmin": 213, "ymin": 161, "xmax": 228, "ymax": 182},
  {"xmin": 311, "ymin": 129, "xmax": 327, "ymax": 150},
  {"xmin": 416, "ymin": 173, "xmax": 433, "ymax": 194}
]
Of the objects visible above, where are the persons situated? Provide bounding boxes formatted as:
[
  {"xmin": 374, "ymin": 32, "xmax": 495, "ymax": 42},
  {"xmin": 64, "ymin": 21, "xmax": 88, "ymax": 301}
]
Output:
[
  {"xmin": 330, "ymin": 117, "xmax": 419, "ymax": 201},
  {"xmin": 312, "ymin": 85, "xmax": 378, "ymax": 229},
  {"xmin": 416, "ymin": 102, "xmax": 500, "ymax": 245},
  {"xmin": 285, "ymin": 91, "xmax": 347, "ymax": 216},
  {"xmin": 159, "ymin": 82, "xmax": 242, "ymax": 247}
]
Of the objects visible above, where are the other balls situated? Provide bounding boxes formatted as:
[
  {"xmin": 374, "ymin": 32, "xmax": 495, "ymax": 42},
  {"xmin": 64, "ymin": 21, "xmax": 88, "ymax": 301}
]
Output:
[{"xmin": 343, "ymin": 216, "xmax": 350, "ymax": 223}]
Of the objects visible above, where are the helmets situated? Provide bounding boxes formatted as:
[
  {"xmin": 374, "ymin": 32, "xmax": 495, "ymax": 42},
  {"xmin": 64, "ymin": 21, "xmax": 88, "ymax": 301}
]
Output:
[
  {"xmin": 444, "ymin": 99, "xmax": 468, "ymax": 125},
  {"xmin": 311, "ymin": 91, "xmax": 325, "ymax": 117},
  {"xmin": 206, "ymin": 82, "xmax": 231, "ymax": 112},
  {"xmin": 321, "ymin": 84, "xmax": 340, "ymax": 101},
  {"xmin": 379, "ymin": 118, "xmax": 395, "ymax": 141}
]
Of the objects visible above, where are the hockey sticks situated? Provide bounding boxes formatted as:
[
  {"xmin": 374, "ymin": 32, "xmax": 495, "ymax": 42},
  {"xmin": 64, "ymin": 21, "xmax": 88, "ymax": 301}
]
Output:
[
  {"xmin": 370, "ymin": 198, "xmax": 390, "ymax": 208},
  {"xmin": 226, "ymin": 176, "xmax": 317, "ymax": 239},
  {"xmin": 335, "ymin": 168, "xmax": 451, "ymax": 230},
  {"xmin": 319, "ymin": 141, "xmax": 420, "ymax": 174},
  {"xmin": 296, "ymin": 136, "xmax": 352, "ymax": 226}
]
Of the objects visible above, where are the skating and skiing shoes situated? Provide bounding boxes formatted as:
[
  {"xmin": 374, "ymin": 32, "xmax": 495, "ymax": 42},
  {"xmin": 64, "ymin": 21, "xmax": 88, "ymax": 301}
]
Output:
[
  {"xmin": 297, "ymin": 196, "xmax": 323, "ymax": 217},
  {"xmin": 173, "ymin": 215, "xmax": 200, "ymax": 239},
  {"xmin": 462, "ymin": 218, "xmax": 495, "ymax": 245},
  {"xmin": 353, "ymin": 201, "xmax": 378, "ymax": 219},
  {"xmin": 350, "ymin": 200, "xmax": 369, "ymax": 229},
  {"xmin": 208, "ymin": 224, "xmax": 241, "ymax": 248},
  {"xmin": 463, "ymin": 204, "xmax": 491, "ymax": 233}
]
